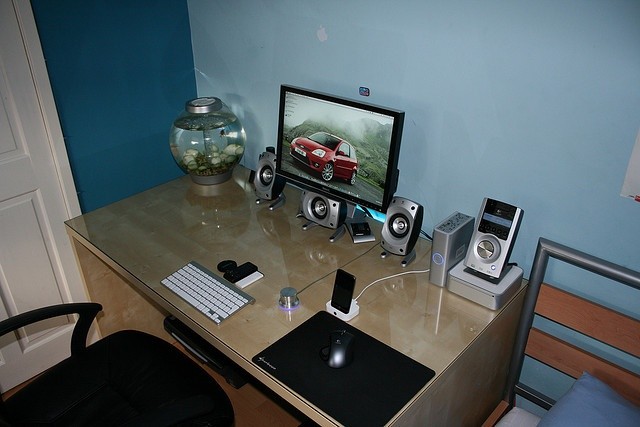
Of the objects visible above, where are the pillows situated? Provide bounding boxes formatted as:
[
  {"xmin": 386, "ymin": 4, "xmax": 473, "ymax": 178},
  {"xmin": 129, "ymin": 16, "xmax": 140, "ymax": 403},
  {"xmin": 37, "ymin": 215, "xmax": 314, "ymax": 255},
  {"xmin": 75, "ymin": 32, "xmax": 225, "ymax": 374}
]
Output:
[{"xmin": 536, "ymin": 372, "xmax": 640, "ymax": 427}]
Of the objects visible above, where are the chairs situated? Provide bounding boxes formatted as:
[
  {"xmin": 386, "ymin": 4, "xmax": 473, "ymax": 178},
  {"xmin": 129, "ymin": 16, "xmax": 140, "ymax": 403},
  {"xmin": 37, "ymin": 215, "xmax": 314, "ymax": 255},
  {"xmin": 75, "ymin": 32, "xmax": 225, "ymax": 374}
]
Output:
[
  {"xmin": 481, "ymin": 237, "xmax": 640, "ymax": 427},
  {"xmin": 0, "ymin": 301, "xmax": 236, "ymax": 427}
]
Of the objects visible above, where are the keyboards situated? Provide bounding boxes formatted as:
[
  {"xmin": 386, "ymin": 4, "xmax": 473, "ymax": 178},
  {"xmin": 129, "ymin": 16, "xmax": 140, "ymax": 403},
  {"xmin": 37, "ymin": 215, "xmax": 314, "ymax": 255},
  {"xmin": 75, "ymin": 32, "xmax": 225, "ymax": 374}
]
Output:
[{"xmin": 160, "ymin": 260, "xmax": 256, "ymax": 326}]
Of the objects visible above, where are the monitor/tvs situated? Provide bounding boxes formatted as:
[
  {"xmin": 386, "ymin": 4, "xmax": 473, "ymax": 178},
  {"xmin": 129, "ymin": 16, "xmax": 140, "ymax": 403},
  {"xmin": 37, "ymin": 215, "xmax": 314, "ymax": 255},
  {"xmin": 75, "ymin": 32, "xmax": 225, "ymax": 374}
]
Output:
[{"xmin": 275, "ymin": 83, "xmax": 405, "ymax": 225}]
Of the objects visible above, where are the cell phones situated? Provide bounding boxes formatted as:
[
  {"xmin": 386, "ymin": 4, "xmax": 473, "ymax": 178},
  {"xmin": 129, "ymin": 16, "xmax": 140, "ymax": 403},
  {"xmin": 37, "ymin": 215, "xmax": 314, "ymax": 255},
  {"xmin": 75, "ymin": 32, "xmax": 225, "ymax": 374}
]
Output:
[
  {"xmin": 331, "ymin": 268, "xmax": 356, "ymax": 314},
  {"xmin": 223, "ymin": 261, "xmax": 258, "ymax": 284}
]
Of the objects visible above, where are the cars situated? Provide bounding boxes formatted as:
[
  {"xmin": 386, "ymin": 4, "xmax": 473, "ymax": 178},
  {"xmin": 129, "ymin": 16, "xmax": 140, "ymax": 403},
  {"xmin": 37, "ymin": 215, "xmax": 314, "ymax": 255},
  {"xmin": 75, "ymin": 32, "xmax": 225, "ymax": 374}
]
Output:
[{"xmin": 290, "ymin": 132, "xmax": 358, "ymax": 186}]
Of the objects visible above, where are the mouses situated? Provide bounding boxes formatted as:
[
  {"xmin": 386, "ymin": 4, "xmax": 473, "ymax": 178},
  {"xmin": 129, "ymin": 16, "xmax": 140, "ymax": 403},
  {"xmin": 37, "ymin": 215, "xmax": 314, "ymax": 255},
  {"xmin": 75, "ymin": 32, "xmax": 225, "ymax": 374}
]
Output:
[{"xmin": 320, "ymin": 329, "xmax": 356, "ymax": 369}]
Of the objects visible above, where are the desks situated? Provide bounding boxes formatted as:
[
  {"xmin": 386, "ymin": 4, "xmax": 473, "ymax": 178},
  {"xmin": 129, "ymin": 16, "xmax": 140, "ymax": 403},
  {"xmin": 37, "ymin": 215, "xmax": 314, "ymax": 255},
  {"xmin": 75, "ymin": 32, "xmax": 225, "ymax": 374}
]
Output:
[{"xmin": 63, "ymin": 162, "xmax": 528, "ymax": 427}]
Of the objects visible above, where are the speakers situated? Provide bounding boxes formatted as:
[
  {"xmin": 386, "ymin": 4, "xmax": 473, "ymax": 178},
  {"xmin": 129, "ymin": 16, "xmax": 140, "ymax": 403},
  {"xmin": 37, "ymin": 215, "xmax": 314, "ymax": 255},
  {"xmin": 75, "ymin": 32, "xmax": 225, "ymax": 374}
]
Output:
[
  {"xmin": 380, "ymin": 196, "xmax": 424, "ymax": 268},
  {"xmin": 253, "ymin": 151, "xmax": 286, "ymax": 211},
  {"xmin": 297, "ymin": 189, "xmax": 348, "ymax": 243}
]
